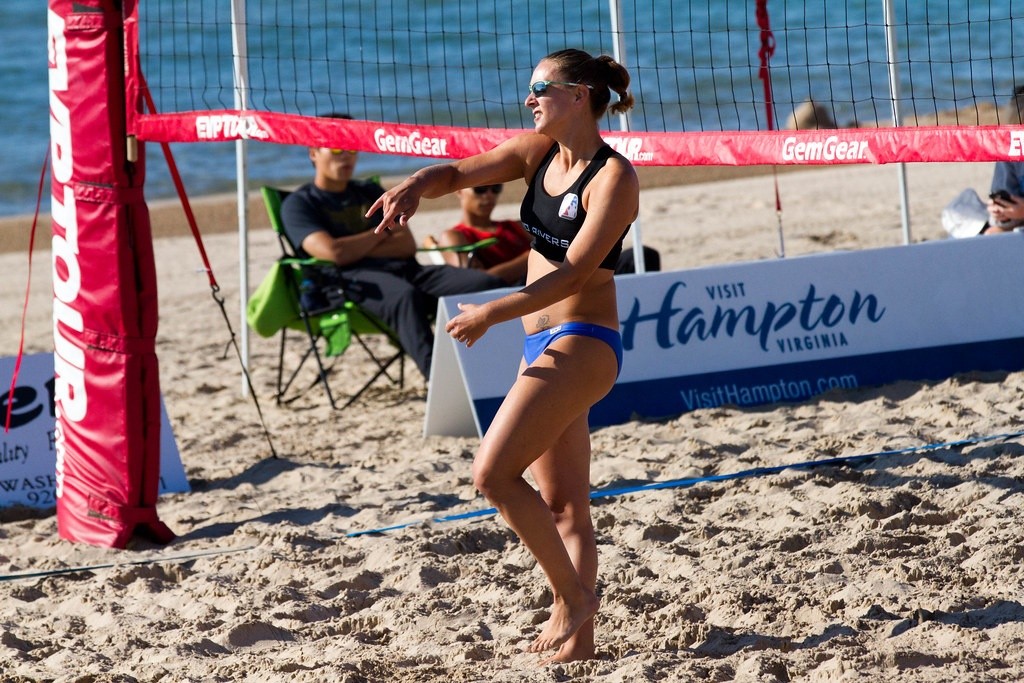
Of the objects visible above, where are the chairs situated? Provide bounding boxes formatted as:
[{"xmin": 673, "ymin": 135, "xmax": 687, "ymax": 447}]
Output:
[
  {"xmin": 247, "ymin": 173, "xmax": 495, "ymax": 409},
  {"xmin": 423, "ymin": 235, "xmax": 458, "ymax": 265}
]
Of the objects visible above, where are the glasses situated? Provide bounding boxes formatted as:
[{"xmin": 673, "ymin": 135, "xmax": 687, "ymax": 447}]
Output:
[
  {"xmin": 528, "ymin": 80, "xmax": 593, "ymax": 98},
  {"xmin": 472, "ymin": 183, "xmax": 503, "ymax": 195},
  {"xmin": 329, "ymin": 149, "xmax": 359, "ymax": 155}
]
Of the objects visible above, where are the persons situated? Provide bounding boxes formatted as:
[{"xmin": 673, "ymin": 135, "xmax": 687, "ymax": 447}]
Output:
[
  {"xmin": 364, "ymin": 49, "xmax": 639, "ymax": 665},
  {"xmin": 940, "ymin": 85, "xmax": 1024, "ymax": 239},
  {"xmin": 440, "ymin": 184, "xmax": 660, "ymax": 287},
  {"xmin": 280, "ymin": 114, "xmax": 513, "ymax": 390}
]
